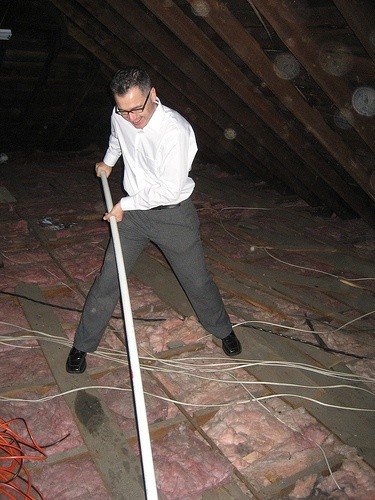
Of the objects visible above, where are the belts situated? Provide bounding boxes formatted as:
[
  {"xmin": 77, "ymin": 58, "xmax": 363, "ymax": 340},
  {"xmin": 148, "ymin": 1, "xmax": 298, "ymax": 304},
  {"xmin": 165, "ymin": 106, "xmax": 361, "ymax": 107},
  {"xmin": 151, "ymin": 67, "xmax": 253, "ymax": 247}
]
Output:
[{"xmin": 150, "ymin": 203, "xmax": 180, "ymax": 210}]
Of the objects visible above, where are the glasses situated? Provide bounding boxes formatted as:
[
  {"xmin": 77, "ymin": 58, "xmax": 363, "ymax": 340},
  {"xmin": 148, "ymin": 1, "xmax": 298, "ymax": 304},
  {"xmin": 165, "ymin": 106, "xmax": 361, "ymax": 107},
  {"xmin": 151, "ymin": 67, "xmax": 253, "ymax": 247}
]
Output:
[{"xmin": 115, "ymin": 89, "xmax": 151, "ymax": 115}]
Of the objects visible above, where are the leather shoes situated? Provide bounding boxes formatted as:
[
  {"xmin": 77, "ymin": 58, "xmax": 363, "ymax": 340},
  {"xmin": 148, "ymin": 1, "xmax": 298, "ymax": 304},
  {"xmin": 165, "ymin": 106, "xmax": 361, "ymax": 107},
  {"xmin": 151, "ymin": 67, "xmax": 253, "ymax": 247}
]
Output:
[
  {"xmin": 222, "ymin": 330, "xmax": 242, "ymax": 356},
  {"xmin": 66, "ymin": 346, "xmax": 86, "ymax": 374}
]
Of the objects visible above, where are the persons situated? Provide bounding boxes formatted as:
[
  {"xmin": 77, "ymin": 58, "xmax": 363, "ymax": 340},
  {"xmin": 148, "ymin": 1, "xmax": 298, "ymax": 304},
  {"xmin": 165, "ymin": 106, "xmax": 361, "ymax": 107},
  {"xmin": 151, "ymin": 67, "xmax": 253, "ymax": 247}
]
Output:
[{"xmin": 65, "ymin": 65, "xmax": 242, "ymax": 374}]
